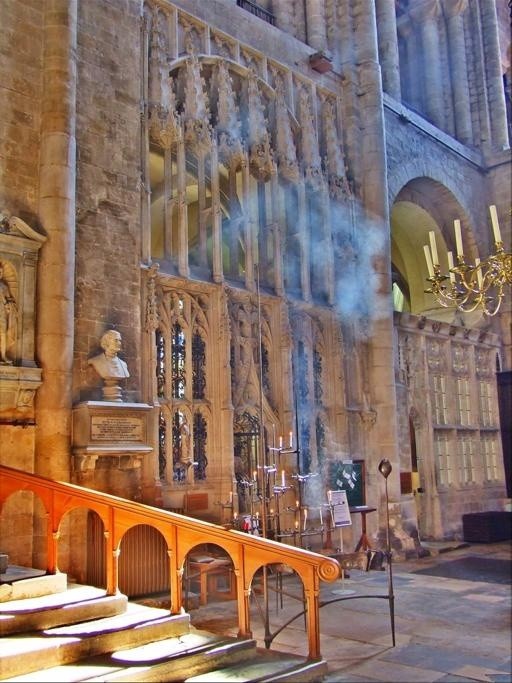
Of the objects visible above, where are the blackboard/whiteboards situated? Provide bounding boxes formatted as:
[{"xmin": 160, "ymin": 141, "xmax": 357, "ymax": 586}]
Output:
[
  {"xmin": 324, "ymin": 459, "xmax": 366, "ymax": 506},
  {"xmin": 327, "ymin": 490, "xmax": 353, "ymax": 528}
]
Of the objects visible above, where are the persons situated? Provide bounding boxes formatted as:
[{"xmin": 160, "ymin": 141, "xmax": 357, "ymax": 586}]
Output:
[{"xmin": 87, "ymin": 330, "xmax": 130, "ymax": 379}]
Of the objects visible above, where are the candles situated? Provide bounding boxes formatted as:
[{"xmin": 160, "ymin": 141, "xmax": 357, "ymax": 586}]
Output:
[
  {"xmin": 489, "ymin": 205, "xmax": 501, "ymax": 242},
  {"xmin": 447, "ymin": 252, "xmax": 455, "ymax": 282},
  {"xmin": 429, "ymin": 231, "xmax": 439, "ymax": 264},
  {"xmin": 229, "ymin": 432, "xmax": 323, "ymax": 530},
  {"xmin": 475, "ymin": 258, "xmax": 483, "ymax": 288},
  {"xmin": 423, "ymin": 245, "xmax": 435, "ymax": 276},
  {"xmin": 454, "ymin": 219, "xmax": 463, "ymax": 255}
]
